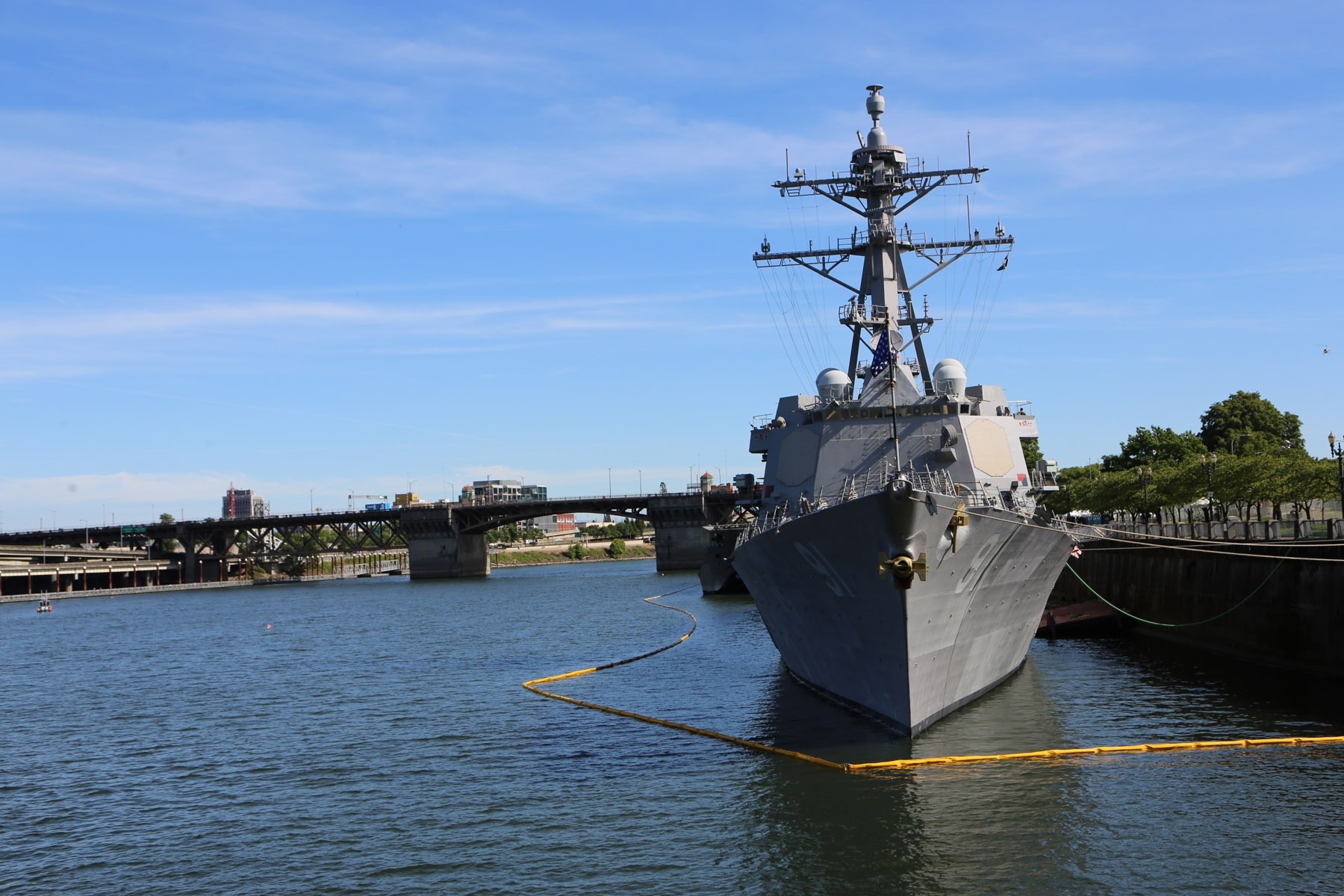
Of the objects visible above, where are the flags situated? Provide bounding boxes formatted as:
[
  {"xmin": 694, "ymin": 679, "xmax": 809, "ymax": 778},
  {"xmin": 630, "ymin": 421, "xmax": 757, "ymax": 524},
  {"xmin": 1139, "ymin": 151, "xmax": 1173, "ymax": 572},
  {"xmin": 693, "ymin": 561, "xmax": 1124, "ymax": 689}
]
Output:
[
  {"xmin": 870, "ymin": 323, "xmax": 899, "ymax": 378},
  {"xmin": 996, "ymin": 255, "xmax": 1008, "ymax": 272}
]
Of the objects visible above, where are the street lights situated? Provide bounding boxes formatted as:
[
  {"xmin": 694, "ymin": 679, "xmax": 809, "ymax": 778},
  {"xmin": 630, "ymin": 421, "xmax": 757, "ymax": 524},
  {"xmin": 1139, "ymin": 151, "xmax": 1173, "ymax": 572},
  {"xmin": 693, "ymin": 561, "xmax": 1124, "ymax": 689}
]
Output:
[
  {"xmin": 79, "ymin": 519, "xmax": 88, "ymax": 549},
  {"xmin": 1137, "ymin": 467, "xmax": 1152, "ymax": 523},
  {"xmin": 1231, "ymin": 434, "xmax": 1253, "ymax": 455},
  {"xmin": 1327, "ymin": 431, "xmax": 1344, "ymax": 520},
  {"xmin": 1199, "ymin": 454, "xmax": 1217, "ymax": 521}
]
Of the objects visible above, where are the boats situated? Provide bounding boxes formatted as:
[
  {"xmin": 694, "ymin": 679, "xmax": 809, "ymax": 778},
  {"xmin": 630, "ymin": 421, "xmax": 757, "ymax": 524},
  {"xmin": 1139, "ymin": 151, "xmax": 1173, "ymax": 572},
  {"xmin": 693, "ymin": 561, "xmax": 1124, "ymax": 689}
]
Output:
[
  {"xmin": 36, "ymin": 589, "xmax": 52, "ymax": 612},
  {"xmin": 692, "ymin": 522, "xmax": 755, "ymax": 595},
  {"xmin": 726, "ymin": 82, "xmax": 1076, "ymax": 739}
]
